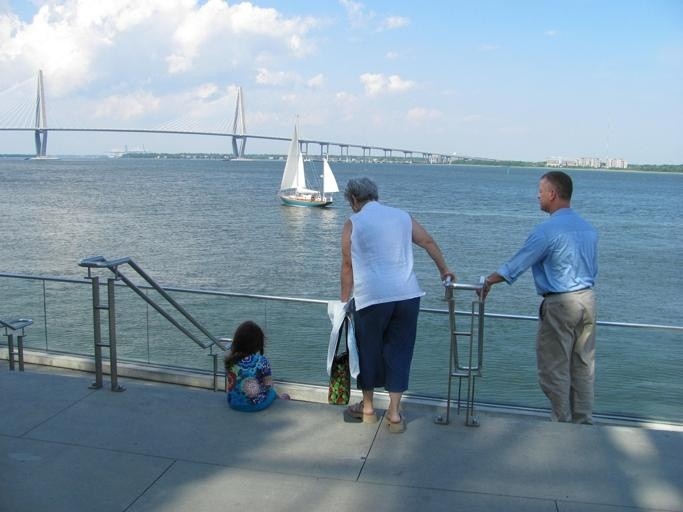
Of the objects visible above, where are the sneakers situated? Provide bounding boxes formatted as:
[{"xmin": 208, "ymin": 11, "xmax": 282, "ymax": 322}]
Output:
[{"xmin": 280, "ymin": 392, "xmax": 290, "ymax": 400}]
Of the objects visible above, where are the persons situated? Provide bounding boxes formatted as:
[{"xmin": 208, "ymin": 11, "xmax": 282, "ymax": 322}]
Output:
[
  {"xmin": 337, "ymin": 176, "xmax": 457, "ymax": 434},
  {"xmin": 220, "ymin": 319, "xmax": 291, "ymax": 413},
  {"xmin": 472, "ymin": 169, "xmax": 600, "ymax": 427}
]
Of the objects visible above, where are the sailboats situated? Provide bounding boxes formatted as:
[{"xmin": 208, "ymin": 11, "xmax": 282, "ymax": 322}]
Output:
[{"xmin": 275, "ymin": 114, "xmax": 340, "ymax": 208}]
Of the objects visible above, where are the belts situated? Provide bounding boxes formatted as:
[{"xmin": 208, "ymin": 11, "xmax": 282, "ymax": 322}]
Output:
[{"xmin": 541, "ymin": 286, "xmax": 592, "ymax": 297}]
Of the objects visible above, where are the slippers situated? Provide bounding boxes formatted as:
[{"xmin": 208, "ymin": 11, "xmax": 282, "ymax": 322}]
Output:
[
  {"xmin": 347, "ymin": 399, "xmax": 377, "ymax": 424},
  {"xmin": 382, "ymin": 408, "xmax": 406, "ymax": 433}
]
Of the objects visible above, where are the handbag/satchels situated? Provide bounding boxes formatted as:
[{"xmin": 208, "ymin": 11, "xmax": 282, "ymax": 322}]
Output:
[{"xmin": 326, "ymin": 315, "xmax": 351, "ymax": 406}]
